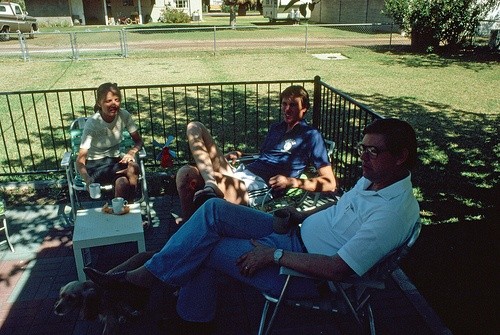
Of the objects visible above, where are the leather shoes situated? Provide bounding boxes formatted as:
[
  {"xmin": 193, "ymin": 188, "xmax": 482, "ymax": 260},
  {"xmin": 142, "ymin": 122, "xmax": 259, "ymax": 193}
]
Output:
[
  {"xmin": 84, "ymin": 267, "xmax": 152, "ymax": 310},
  {"xmin": 155, "ymin": 312, "xmax": 216, "ymax": 335}
]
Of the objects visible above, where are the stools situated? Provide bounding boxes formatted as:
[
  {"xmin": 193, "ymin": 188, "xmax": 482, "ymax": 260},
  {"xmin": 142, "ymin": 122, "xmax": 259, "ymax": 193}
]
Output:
[{"xmin": 0, "ymin": 200, "xmax": 14, "ymax": 252}]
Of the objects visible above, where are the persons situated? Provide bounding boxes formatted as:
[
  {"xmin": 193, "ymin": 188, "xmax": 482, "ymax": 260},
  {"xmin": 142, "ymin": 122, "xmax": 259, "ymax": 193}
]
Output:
[
  {"xmin": 75, "ymin": 83, "xmax": 143, "ymax": 206},
  {"xmin": 84, "ymin": 119, "xmax": 420, "ymax": 335},
  {"xmin": 176, "ymin": 85, "xmax": 336, "ymax": 217}
]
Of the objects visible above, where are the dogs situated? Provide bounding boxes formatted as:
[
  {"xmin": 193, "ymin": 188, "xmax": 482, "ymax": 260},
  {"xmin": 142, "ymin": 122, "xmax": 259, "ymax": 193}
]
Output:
[{"xmin": 54, "ymin": 252, "xmax": 165, "ymax": 335}]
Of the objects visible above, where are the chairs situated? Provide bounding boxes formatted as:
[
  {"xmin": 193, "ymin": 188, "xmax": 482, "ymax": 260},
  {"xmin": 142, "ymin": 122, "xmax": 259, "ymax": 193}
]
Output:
[
  {"xmin": 60, "ymin": 117, "xmax": 150, "ymax": 225},
  {"xmin": 225, "ymin": 140, "xmax": 336, "ymax": 215},
  {"xmin": 257, "ymin": 220, "xmax": 423, "ymax": 335}
]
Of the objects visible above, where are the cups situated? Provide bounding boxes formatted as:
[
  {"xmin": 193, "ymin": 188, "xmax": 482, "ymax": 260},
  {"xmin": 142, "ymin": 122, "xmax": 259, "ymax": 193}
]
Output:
[
  {"xmin": 89, "ymin": 183, "xmax": 101, "ymax": 199},
  {"xmin": 273, "ymin": 209, "xmax": 291, "ymax": 235},
  {"xmin": 112, "ymin": 197, "xmax": 128, "ymax": 214}
]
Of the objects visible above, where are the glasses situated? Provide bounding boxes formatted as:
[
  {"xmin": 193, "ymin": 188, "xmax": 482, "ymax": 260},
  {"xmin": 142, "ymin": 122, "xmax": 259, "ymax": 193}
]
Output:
[{"xmin": 356, "ymin": 144, "xmax": 395, "ymax": 159}]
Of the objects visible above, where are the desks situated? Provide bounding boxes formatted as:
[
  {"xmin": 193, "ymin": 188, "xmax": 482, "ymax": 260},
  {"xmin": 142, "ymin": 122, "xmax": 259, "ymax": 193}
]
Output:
[{"xmin": 74, "ymin": 203, "xmax": 145, "ymax": 283}]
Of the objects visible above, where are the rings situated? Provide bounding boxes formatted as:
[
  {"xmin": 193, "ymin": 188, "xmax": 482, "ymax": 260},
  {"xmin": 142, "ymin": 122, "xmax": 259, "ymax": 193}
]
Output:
[{"xmin": 245, "ymin": 266, "xmax": 249, "ymax": 270}]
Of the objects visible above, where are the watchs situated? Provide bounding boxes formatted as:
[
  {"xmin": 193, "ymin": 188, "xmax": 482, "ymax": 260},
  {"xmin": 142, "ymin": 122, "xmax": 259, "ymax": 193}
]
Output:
[{"xmin": 273, "ymin": 249, "xmax": 283, "ymax": 265}]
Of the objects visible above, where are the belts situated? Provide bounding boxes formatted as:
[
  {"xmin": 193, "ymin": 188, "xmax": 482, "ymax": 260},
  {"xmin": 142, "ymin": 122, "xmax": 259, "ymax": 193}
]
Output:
[{"xmin": 295, "ymin": 226, "xmax": 307, "ymax": 254}]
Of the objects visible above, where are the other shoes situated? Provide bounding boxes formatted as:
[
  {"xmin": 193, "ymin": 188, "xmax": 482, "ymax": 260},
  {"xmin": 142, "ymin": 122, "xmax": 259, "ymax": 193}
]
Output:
[{"xmin": 192, "ymin": 182, "xmax": 224, "ymax": 204}]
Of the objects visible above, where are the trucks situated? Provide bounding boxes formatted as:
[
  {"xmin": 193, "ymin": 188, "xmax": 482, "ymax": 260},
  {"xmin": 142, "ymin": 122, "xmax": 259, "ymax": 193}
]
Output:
[{"xmin": 263, "ymin": 0, "xmax": 313, "ymax": 24}]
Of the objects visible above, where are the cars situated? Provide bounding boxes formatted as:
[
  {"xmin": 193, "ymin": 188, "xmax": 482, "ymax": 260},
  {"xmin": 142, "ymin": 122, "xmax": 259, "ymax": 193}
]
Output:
[{"xmin": 0, "ymin": 1, "xmax": 38, "ymax": 42}]
